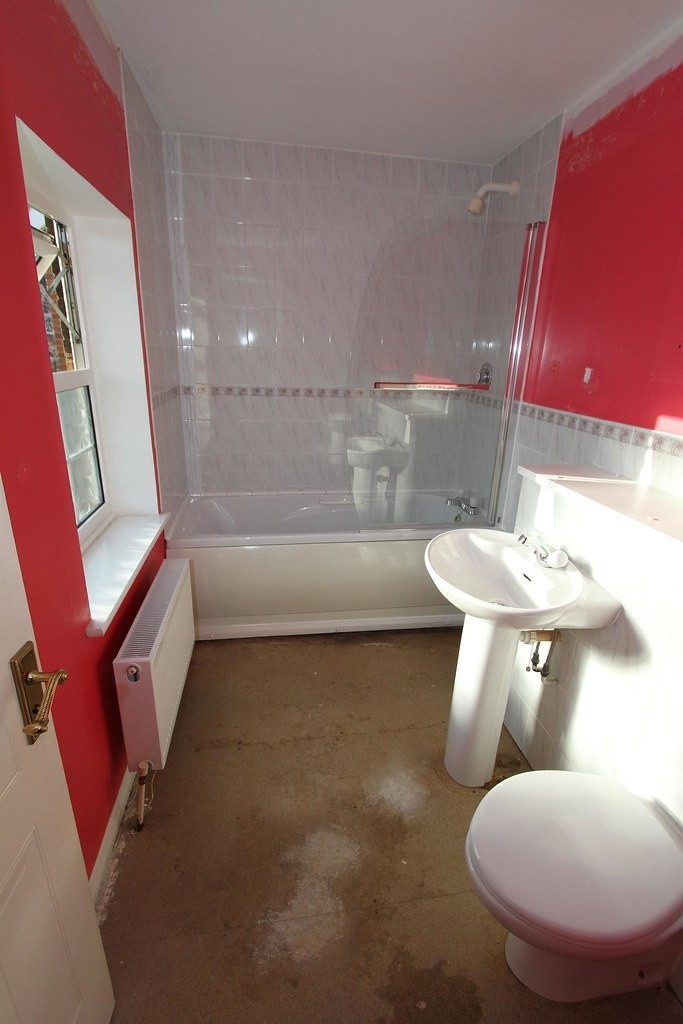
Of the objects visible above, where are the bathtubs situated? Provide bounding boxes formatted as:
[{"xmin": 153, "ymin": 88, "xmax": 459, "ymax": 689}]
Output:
[{"xmin": 165, "ymin": 472, "xmax": 499, "ymax": 639}]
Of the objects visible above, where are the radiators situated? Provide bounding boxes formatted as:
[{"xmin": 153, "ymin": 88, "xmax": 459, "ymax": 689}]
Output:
[{"xmin": 113, "ymin": 558, "xmax": 196, "ymax": 772}]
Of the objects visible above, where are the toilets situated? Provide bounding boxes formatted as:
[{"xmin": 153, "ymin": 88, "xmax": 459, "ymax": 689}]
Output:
[
  {"xmin": 324, "ymin": 413, "xmax": 371, "ymax": 464},
  {"xmin": 459, "ymin": 766, "xmax": 683, "ymax": 1004}
]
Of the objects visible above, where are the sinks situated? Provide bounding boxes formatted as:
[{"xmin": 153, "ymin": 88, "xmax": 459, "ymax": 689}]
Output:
[
  {"xmin": 343, "ymin": 434, "xmax": 410, "ymax": 475},
  {"xmin": 423, "ymin": 523, "xmax": 624, "ymax": 637}
]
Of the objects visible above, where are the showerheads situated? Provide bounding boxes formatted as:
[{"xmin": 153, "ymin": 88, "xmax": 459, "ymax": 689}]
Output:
[{"xmin": 467, "ymin": 196, "xmax": 484, "ymax": 216}]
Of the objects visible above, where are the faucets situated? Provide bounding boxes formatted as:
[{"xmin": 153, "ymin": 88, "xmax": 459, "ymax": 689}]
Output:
[
  {"xmin": 516, "ymin": 533, "xmax": 568, "ymax": 567},
  {"xmin": 444, "ymin": 492, "xmax": 483, "ymax": 517}
]
